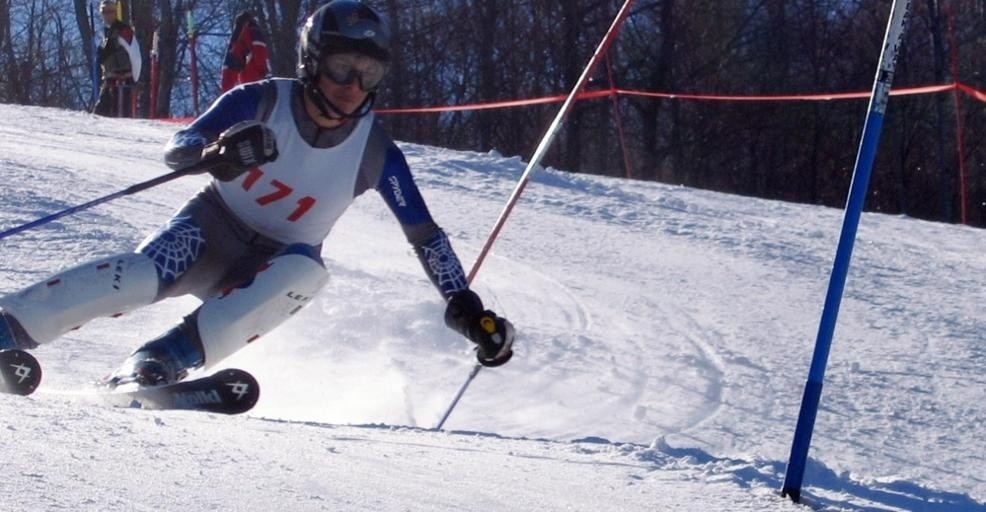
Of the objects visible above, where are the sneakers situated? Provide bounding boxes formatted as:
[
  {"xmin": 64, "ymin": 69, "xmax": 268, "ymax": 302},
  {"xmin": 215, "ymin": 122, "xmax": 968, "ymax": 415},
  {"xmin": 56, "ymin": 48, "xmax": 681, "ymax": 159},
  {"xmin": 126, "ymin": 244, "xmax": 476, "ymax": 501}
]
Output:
[{"xmin": 127, "ymin": 328, "xmax": 202, "ymax": 386}]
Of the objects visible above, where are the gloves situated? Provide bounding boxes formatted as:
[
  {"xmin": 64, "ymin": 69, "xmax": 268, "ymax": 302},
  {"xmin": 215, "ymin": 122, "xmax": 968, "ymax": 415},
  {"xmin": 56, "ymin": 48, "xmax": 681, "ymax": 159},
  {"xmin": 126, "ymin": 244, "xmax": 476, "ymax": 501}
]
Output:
[
  {"xmin": 196, "ymin": 119, "xmax": 278, "ymax": 183},
  {"xmin": 442, "ymin": 288, "xmax": 517, "ymax": 363}
]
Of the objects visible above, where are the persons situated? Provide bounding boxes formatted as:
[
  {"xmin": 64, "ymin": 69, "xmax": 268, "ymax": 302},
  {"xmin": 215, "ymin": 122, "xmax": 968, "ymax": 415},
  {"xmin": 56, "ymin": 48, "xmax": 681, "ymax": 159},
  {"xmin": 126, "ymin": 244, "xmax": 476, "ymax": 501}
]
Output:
[
  {"xmin": 222, "ymin": 12, "xmax": 274, "ymax": 102},
  {"xmin": 0, "ymin": 1, "xmax": 520, "ymax": 414},
  {"xmin": 92, "ymin": 1, "xmax": 143, "ymax": 117}
]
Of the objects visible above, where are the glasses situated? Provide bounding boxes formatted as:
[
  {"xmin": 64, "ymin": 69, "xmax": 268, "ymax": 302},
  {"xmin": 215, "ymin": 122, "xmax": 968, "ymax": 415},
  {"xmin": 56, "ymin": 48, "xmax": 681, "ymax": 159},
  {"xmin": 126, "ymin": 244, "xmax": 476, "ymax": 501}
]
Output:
[{"xmin": 318, "ymin": 51, "xmax": 390, "ymax": 91}]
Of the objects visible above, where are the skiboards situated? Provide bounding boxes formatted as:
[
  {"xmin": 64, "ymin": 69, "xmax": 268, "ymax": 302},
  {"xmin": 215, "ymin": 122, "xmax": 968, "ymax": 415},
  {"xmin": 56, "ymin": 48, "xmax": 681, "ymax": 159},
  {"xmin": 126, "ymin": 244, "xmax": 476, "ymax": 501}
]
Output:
[{"xmin": 0, "ymin": 348, "xmax": 259, "ymax": 414}]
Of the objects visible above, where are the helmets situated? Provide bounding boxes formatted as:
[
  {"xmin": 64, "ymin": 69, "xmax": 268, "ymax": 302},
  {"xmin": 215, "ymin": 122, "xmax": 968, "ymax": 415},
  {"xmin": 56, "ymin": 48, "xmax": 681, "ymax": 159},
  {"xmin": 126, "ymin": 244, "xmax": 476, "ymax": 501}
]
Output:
[
  {"xmin": 99, "ymin": 0, "xmax": 117, "ymax": 14},
  {"xmin": 295, "ymin": 1, "xmax": 394, "ymax": 91}
]
